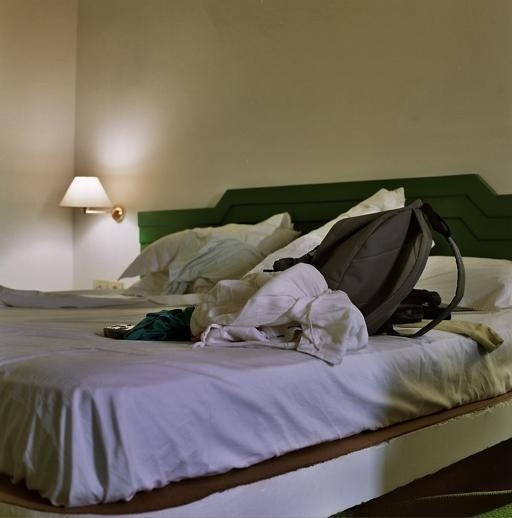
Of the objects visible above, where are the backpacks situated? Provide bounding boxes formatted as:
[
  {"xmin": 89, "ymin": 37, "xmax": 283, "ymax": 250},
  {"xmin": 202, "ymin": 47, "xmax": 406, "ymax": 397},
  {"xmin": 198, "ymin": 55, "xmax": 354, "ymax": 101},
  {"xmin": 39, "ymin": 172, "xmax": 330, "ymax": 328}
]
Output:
[{"xmin": 263, "ymin": 198, "xmax": 467, "ymax": 338}]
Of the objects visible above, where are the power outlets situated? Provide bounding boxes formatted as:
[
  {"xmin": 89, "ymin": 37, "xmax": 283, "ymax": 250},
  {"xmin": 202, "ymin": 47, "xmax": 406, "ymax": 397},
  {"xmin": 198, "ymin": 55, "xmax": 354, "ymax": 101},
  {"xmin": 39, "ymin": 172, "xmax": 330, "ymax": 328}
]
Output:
[
  {"xmin": 91, "ymin": 279, "xmax": 109, "ymax": 290},
  {"xmin": 107, "ymin": 282, "xmax": 124, "ymax": 291}
]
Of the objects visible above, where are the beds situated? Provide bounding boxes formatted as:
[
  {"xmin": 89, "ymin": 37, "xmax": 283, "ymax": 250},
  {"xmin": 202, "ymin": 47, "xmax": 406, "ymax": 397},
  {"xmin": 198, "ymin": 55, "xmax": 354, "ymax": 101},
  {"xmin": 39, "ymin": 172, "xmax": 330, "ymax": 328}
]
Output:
[{"xmin": 0, "ymin": 171, "xmax": 511, "ymax": 517}]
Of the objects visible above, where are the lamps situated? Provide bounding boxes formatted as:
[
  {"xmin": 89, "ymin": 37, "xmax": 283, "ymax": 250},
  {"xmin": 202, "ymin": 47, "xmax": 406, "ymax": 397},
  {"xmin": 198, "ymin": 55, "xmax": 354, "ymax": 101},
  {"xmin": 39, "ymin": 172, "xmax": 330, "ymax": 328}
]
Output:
[{"xmin": 59, "ymin": 173, "xmax": 126, "ymax": 223}]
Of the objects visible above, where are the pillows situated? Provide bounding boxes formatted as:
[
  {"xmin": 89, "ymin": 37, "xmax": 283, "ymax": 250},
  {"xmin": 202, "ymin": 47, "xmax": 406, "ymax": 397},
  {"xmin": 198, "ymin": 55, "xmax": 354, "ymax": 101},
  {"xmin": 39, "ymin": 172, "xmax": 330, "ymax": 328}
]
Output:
[
  {"xmin": 418, "ymin": 252, "xmax": 512, "ymax": 313},
  {"xmin": 118, "ymin": 181, "xmax": 406, "ymax": 294}
]
